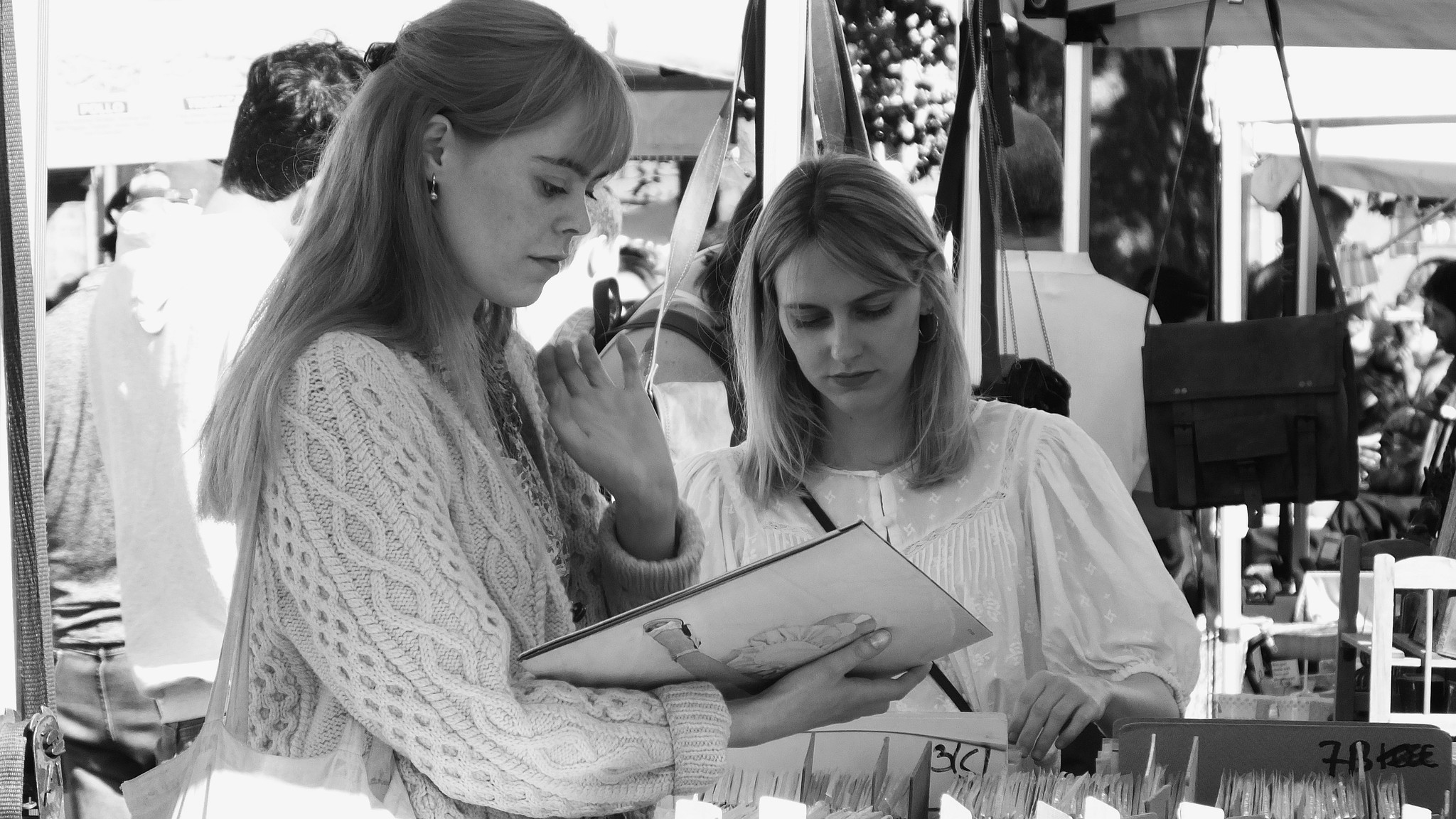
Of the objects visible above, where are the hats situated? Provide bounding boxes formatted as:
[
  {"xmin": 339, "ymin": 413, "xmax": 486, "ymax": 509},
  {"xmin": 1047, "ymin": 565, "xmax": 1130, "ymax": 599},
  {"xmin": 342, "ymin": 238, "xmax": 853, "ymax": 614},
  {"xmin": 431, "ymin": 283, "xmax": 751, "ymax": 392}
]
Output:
[{"xmin": 1420, "ymin": 261, "xmax": 1455, "ymax": 315}]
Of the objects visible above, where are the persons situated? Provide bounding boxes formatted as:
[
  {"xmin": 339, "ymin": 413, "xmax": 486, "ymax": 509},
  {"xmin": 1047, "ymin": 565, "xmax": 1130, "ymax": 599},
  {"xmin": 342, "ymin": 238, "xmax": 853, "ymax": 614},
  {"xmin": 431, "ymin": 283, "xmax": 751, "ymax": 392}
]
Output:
[{"xmin": 42, "ymin": 3, "xmax": 1456, "ymax": 819}]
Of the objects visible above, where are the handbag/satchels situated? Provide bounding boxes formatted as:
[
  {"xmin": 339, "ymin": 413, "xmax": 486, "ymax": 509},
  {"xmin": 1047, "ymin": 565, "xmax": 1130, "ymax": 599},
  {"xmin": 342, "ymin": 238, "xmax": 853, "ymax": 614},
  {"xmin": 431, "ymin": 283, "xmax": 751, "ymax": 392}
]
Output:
[
  {"xmin": 978, "ymin": 354, "xmax": 1072, "ymax": 418},
  {"xmin": 1140, "ymin": 309, "xmax": 1363, "ymax": 509}
]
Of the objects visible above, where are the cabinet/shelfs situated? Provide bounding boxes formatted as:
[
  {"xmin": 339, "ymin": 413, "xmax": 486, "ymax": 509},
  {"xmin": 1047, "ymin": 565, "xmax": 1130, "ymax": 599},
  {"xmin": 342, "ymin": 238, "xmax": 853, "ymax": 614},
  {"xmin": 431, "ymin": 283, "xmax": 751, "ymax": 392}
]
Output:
[{"xmin": 1332, "ymin": 534, "xmax": 1456, "ymax": 740}]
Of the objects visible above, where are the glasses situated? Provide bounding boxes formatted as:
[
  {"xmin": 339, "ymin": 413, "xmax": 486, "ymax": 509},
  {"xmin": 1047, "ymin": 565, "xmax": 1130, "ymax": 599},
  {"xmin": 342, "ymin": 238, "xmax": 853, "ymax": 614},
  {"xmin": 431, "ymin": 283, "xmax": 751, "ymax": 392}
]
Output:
[{"xmin": 1423, "ymin": 306, "xmax": 1446, "ymax": 320}]
